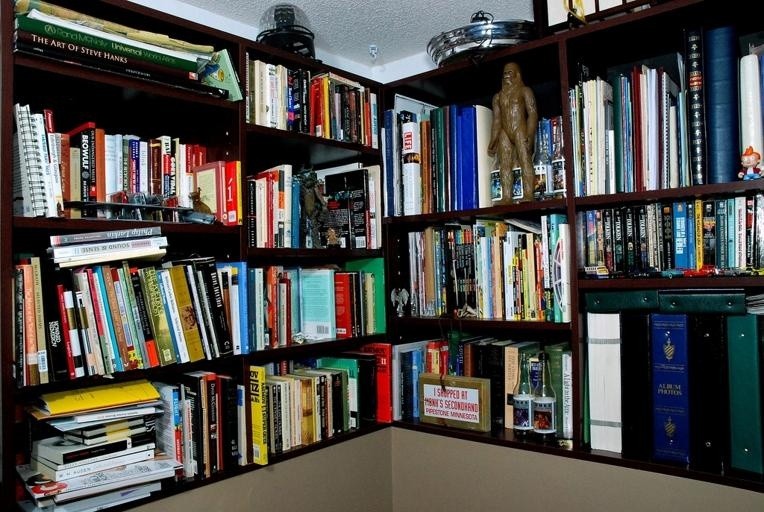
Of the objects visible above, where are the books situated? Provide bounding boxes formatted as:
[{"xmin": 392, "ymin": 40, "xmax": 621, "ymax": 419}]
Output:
[
  {"xmin": 10, "ymin": 1, "xmax": 248, "ymax": 512},
  {"xmin": 381, "ymin": 93, "xmax": 575, "ymax": 453},
  {"xmin": 243, "ymin": 48, "xmax": 404, "ymax": 469},
  {"xmin": 568, "ymin": 16, "xmax": 764, "ymax": 474}
]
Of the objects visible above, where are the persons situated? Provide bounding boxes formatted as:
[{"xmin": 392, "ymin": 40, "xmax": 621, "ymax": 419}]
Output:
[{"xmin": 486, "ymin": 62, "xmax": 538, "ymax": 206}]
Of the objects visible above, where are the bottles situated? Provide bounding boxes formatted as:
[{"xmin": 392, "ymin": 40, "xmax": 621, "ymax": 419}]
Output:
[
  {"xmin": 532, "ymin": 351, "xmax": 558, "ymax": 443},
  {"xmin": 512, "ymin": 353, "xmax": 535, "ymax": 441},
  {"xmin": 488, "ymin": 117, "xmax": 567, "ymax": 201}
]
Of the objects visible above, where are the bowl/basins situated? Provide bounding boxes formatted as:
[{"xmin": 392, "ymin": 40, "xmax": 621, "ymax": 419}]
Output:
[{"xmin": 426, "ymin": 16, "xmax": 535, "ymax": 68}]
